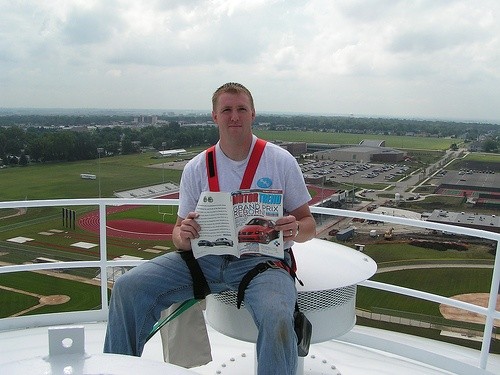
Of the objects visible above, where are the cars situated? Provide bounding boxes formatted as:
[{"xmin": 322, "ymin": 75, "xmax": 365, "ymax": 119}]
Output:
[
  {"xmin": 297, "ymin": 159, "xmax": 408, "ymax": 180},
  {"xmin": 237, "ymin": 216, "xmax": 281, "ymax": 244},
  {"xmin": 198, "ymin": 239, "xmax": 214, "ymax": 247},
  {"xmin": 212, "ymin": 237, "xmax": 234, "ymax": 246}
]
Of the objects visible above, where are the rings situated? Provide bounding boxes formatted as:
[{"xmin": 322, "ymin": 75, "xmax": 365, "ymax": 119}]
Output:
[{"xmin": 289, "ymin": 229, "xmax": 292, "ymax": 236}]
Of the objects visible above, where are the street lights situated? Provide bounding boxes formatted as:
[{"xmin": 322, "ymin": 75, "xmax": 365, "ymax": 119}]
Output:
[
  {"xmin": 162, "ymin": 141, "xmax": 167, "ymax": 182},
  {"xmin": 97, "ymin": 147, "xmax": 103, "ymax": 198}
]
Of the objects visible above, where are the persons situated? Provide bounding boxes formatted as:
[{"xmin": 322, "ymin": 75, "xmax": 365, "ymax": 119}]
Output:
[{"xmin": 103, "ymin": 81, "xmax": 317, "ymax": 375}]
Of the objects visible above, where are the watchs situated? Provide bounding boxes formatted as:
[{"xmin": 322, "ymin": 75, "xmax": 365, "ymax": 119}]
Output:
[{"xmin": 294, "ymin": 222, "xmax": 299, "ymax": 238}]
